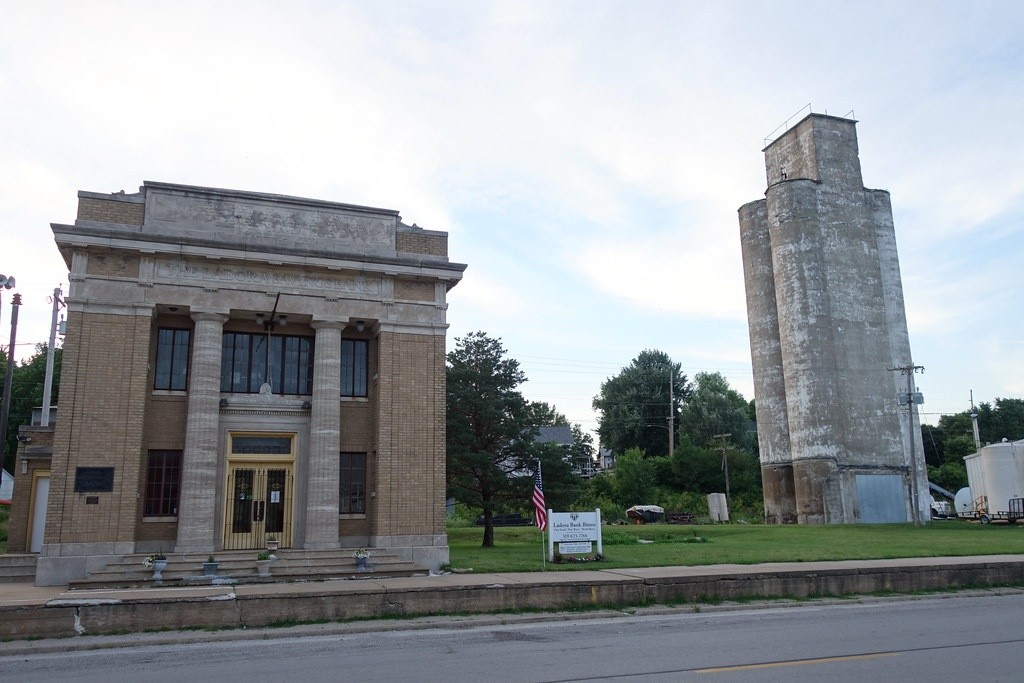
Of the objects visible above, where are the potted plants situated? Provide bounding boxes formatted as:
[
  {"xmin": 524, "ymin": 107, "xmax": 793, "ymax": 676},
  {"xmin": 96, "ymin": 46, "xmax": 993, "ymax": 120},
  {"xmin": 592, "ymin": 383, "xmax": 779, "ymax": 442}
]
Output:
[
  {"xmin": 265, "ymin": 535, "xmax": 280, "ymax": 557},
  {"xmin": 202, "ymin": 554, "xmax": 220, "ymax": 578},
  {"xmin": 255, "ymin": 550, "xmax": 272, "ymax": 574}
]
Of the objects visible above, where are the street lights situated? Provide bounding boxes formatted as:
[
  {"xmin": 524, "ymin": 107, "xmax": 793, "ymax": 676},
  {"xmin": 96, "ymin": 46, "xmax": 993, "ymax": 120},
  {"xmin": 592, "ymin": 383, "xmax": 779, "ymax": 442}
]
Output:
[{"xmin": 647, "ymin": 423, "xmax": 675, "ymax": 456}]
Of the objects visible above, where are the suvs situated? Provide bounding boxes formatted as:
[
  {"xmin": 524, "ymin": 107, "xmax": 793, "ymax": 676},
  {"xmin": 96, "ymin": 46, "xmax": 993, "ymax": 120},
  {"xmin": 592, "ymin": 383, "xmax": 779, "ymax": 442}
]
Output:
[{"xmin": 930, "ymin": 495, "xmax": 951, "ymax": 518}]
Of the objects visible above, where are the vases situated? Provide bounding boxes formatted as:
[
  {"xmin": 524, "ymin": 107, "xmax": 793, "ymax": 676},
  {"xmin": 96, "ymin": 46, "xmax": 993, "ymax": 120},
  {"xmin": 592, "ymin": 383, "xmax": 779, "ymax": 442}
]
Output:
[
  {"xmin": 353, "ymin": 558, "xmax": 369, "ymax": 571},
  {"xmin": 152, "ymin": 559, "xmax": 168, "ymax": 579}
]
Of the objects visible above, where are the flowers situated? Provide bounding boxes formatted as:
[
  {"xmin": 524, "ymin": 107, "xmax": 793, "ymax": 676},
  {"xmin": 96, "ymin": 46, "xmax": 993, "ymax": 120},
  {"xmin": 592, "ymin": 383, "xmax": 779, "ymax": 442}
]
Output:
[
  {"xmin": 141, "ymin": 545, "xmax": 167, "ymax": 568},
  {"xmin": 352, "ymin": 547, "xmax": 370, "ymax": 559}
]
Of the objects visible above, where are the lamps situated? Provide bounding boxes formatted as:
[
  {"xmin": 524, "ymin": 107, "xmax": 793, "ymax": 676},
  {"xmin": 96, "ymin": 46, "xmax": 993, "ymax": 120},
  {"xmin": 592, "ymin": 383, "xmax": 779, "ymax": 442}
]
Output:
[
  {"xmin": 256, "ymin": 313, "xmax": 265, "ymax": 325},
  {"xmin": 356, "ymin": 320, "xmax": 365, "ymax": 332},
  {"xmin": 278, "ymin": 315, "xmax": 288, "ymax": 327}
]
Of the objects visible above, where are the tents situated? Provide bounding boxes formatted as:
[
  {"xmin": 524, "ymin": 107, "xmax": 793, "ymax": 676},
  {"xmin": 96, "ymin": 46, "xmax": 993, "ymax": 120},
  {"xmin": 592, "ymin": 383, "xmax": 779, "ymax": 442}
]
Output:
[{"xmin": 625, "ymin": 505, "xmax": 665, "ymax": 523}]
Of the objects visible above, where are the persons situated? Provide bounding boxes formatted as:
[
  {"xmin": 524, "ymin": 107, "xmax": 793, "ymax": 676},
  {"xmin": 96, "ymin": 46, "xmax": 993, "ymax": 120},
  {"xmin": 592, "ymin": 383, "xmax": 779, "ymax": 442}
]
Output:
[{"xmin": 636, "ymin": 518, "xmax": 640, "ymax": 525}]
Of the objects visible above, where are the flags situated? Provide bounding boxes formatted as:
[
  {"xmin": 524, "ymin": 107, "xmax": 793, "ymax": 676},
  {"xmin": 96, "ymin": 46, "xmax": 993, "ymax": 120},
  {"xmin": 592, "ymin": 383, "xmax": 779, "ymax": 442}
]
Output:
[{"xmin": 531, "ymin": 466, "xmax": 548, "ymax": 532}]
